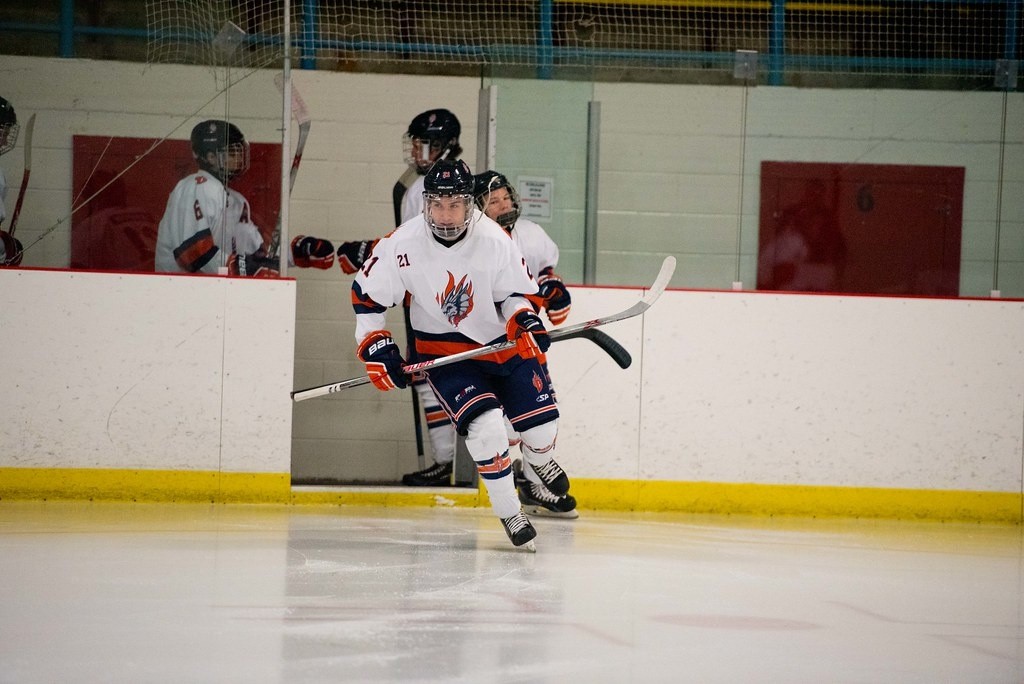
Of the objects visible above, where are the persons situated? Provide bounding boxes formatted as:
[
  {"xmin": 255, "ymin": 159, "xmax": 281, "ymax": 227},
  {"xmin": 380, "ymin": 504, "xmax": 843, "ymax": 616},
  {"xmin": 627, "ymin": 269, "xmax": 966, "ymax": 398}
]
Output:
[
  {"xmin": 0, "ymin": 96, "xmax": 26, "ymax": 267},
  {"xmin": 473, "ymin": 170, "xmax": 581, "ymax": 520},
  {"xmin": 350, "ymin": 160, "xmax": 571, "ymax": 553},
  {"xmin": 154, "ymin": 119, "xmax": 335, "ymax": 278},
  {"xmin": 337, "ymin": 107, "xmax": 463, "ymax": 486}
]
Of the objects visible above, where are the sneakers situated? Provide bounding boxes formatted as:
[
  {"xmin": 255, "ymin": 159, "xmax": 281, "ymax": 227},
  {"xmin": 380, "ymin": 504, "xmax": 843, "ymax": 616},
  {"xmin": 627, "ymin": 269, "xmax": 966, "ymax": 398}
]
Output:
[
  {"xmin": 501, "ymin": 511, "xmax": 537, "ymax": 553},
  {"xmin": 402, "ymin": 459, "xmax": 453, "ymax": 486},
  {"xmin": 519, "ymin": 441, "xmax": 570, "ymax": 500},
  {"xmin": 516, "ymin": 471, "xmax": 580, "ymax": 518}
]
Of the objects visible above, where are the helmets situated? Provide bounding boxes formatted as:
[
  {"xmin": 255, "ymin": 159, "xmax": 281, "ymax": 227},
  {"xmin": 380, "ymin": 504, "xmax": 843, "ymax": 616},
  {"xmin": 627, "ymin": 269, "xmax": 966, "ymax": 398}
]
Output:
[
  {"xmin": 402, "ymin": 108, "xmax": 463, "ymax": 175},
  {"xmin": 190, "ymin": 119, "xmax": 249, "ymax": 185},
  {"xmin": 0, "ymin": 96, "xmax": 20, "ymax": 155},
  {"xmin": 423, "ymin": 158, "xmax": 474, "ymax": 240},
  {"xmin": 473, "ymin": 168, "xmax": 522, "ymax": 228}
]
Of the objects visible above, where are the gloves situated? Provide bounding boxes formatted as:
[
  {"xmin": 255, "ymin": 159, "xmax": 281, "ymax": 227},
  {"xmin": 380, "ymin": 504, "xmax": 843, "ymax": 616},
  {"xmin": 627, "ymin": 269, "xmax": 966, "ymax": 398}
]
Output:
[
  {"xmin": 537, "ymin": 274, "xmax": 570, "ymax": 325},
  {"xmin": 337, "ymin": 235, "xmax": 372, "ymax": 275},
  {"xmin": 356, "ymin": 329, "xmax": 414, "ymax": 391},
  {"xmin": 290, "ymin": 235, "xmax": 334, "ymax": 269},
  {"xmin": 226, "ymin": 253, "xmax": 279, "ymax": 280},
  {"xmin": 0, "ymin": 228, "xmax": 23, "ymax": 267},
  {"xmin": 506, "ymin": 308, "xmax": 551, "ymax": 359}
]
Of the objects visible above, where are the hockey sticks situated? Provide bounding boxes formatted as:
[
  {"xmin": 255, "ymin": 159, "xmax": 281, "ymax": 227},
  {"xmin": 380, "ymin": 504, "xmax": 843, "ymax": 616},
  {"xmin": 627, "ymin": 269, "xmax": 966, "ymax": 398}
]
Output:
[
  {"xmin": 547, "ymin": 325, "xmax": 634, "ymax": 371},
  {"xmin": 266, "ymin": 70, "xmax": 313, "ymax": 261},
  {"xmin": 288, "ymin": 253, "xmax": 678, "ymax": 405}
]
